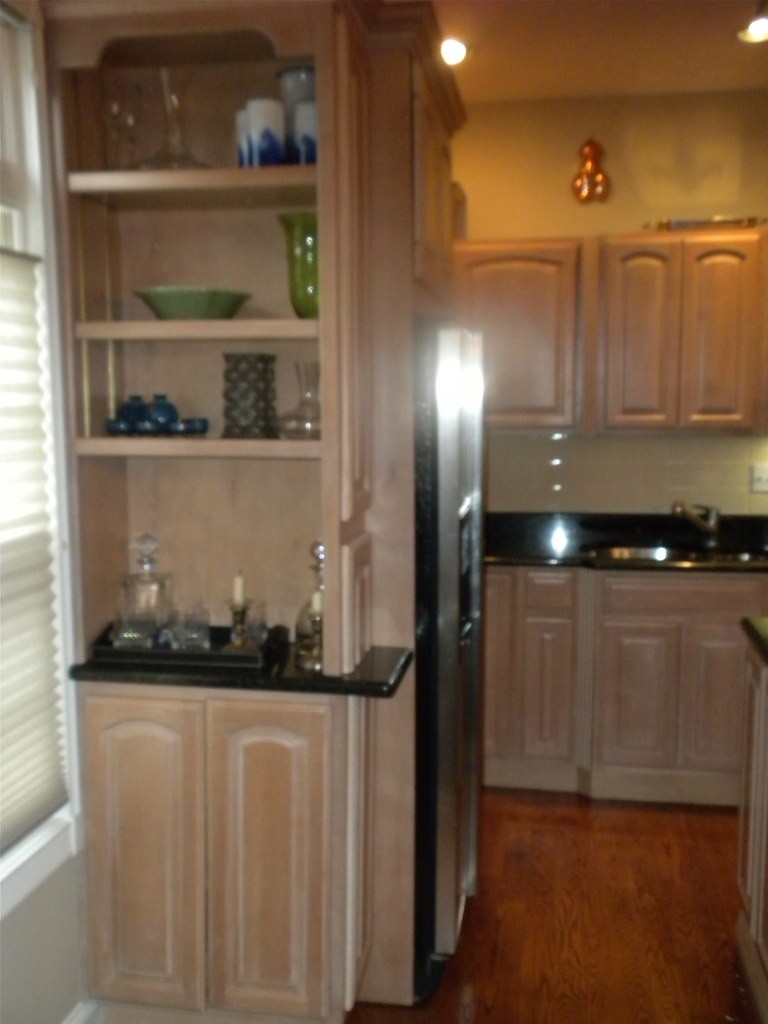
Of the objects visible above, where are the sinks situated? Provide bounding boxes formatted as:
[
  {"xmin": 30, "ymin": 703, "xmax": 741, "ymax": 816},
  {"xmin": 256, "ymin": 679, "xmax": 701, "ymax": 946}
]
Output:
[{"xmin": 588, "ymin": 548, "xmax": 768, "ymax": 568}]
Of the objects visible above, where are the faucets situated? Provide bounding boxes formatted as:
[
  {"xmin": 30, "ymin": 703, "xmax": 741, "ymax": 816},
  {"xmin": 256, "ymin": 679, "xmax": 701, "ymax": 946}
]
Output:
[{"xmin": 671, "ymin": 499, "xmax": 723, "ymax": 554}]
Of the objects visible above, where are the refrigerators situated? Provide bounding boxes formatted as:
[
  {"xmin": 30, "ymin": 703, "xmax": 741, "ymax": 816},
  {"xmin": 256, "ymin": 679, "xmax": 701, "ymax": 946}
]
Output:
[{"xmin": 416, "ymin": 315, "xmax": 484, "ymax": 956}]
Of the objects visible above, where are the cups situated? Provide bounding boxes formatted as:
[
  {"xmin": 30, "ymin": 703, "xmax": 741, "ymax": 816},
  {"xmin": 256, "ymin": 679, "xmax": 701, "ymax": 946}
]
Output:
[
  {"xmin": 276, "ymin": 210, "xmax": 319, "ymax": 319},
  {"xmin": 232, "ymin": 57, "xmax": 317, "ymax": 168},
  {"xmin": 165, "ymin": 598, "xmax": 211, "ymax": 654}
]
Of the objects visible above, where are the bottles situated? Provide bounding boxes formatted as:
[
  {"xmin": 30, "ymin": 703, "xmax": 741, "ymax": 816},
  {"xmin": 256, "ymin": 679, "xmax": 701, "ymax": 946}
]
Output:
[
  {"xmin": 117, "ymin": 535, "xmax": 170, "ymax": 648},
  {"xmin": 104, "ymin": 393, "xmax": 208, "ymax": 438},
  {"xmin": 293, "ymin": 543, "xmax": 326, "ymax": 674},
  {"xmin": 271, "ymin": 360, "xmax": 322, "ymax": 440}
]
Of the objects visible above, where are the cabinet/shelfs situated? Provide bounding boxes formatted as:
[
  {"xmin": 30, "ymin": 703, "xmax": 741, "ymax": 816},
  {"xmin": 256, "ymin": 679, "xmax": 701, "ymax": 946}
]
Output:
[
  {"xmin": 54, "ymin": 0, "xmax": 376, "ymax": 675},
  {"xmin": 452, "ymin": 235, "xmax": 593, "ymax": 439},
  {"xmin": 728, "ymin": 614, "xmax": 768, "ymax": 1024},
  {"xmin": 70, "ymin": 645, "xmax": 416, "ymax": 1024},
  {"xmin": 483, "ymin": 511, "xmax": 768, "ymax": 810},
  {"xmin": 587, "ymin": 225, "xmax": 768, "ymax": 439}
]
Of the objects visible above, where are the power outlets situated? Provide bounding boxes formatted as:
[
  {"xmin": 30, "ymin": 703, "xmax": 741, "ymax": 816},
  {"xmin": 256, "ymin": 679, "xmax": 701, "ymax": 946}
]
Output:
[{"xmin": 752, "ymin": 463, "xmax": 768, "ymax": 493}]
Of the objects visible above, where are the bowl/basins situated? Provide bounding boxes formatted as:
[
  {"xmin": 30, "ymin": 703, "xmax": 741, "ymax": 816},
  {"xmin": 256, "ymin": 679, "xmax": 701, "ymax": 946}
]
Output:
[{"xmin": 134, "ymin": 284, "xmax": 251, "ymax": 319}]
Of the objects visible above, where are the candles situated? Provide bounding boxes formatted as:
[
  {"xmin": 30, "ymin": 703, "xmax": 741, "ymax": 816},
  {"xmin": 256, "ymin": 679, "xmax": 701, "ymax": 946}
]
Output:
[{"xmin": 232, "ymin": 568, "xmax": 247, "ymax": 604}]
